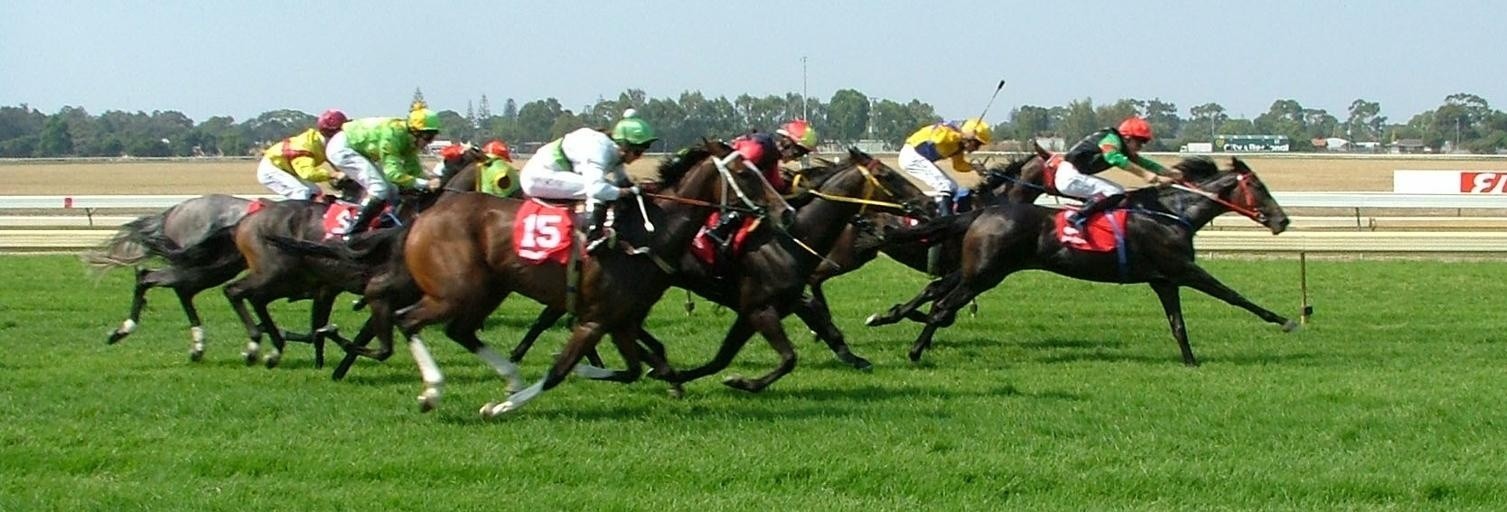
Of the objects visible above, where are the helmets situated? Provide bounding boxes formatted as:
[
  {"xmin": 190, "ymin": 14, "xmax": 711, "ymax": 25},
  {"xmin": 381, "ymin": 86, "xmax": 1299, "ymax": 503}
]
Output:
[
  {"xmin": 408, "ymin": 102, "xmax": 440, "ymax": 134},
  {"xmin": 960, "ymin": 119, "xmax": 992, "ymax": 146},
  {"xmin": 776, "ymin": 119, "xmax": 819, "ymax": 151},
  {"xmin": 611, "ymin": 109, "xmax": 659, "ymax": 146},
  {"xmin": 1117, "ymin": 118, "xmax": 1152, "ymax": 140},
  {"xmin": 317, "ymin": 110, "xmax": 347, "ymax": 133}
]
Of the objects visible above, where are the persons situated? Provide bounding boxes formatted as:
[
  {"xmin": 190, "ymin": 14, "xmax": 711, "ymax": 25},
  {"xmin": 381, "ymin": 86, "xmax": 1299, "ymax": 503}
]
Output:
[
  {"xmin": 430, "ymin": 140, "xmax": 512, "ymax": 177},
  {"xmin": 255, "ymin": 109, "xmax": 355, "ymax": 200},
  {"xmin": 324, "ymin": 107, "xmax": 441, "ymax": 239},
  {"xmin": 897, "ymin": 117, "xmax": 992, "ymax": 217},
  {"xmin": 519, "ymin": 117, "xmax": 658, "ymax": 252},
  {"xmin": 686, "ymin": 121, "xmax": 822, "ymax": 264},
  {"xmin": 1053, "ymin": 118, "xmax": 1184, "ymax": 234}
]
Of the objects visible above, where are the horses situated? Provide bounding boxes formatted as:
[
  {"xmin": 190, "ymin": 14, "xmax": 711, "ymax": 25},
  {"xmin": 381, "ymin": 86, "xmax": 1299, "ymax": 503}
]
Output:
[
  {"xmin": 852, "ymin": 155, "xmax": 1296, "ymax": 368},
  {"xmin": 76, "ymin": 140, "xmax": 1057, "ymax": 420}
]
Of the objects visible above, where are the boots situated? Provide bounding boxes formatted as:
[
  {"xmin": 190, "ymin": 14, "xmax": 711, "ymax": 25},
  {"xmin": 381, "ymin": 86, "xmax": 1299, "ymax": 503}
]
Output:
[{"xmin": 1067, "ymin": 192, "xmax": 1108, "ymax": 240}]
